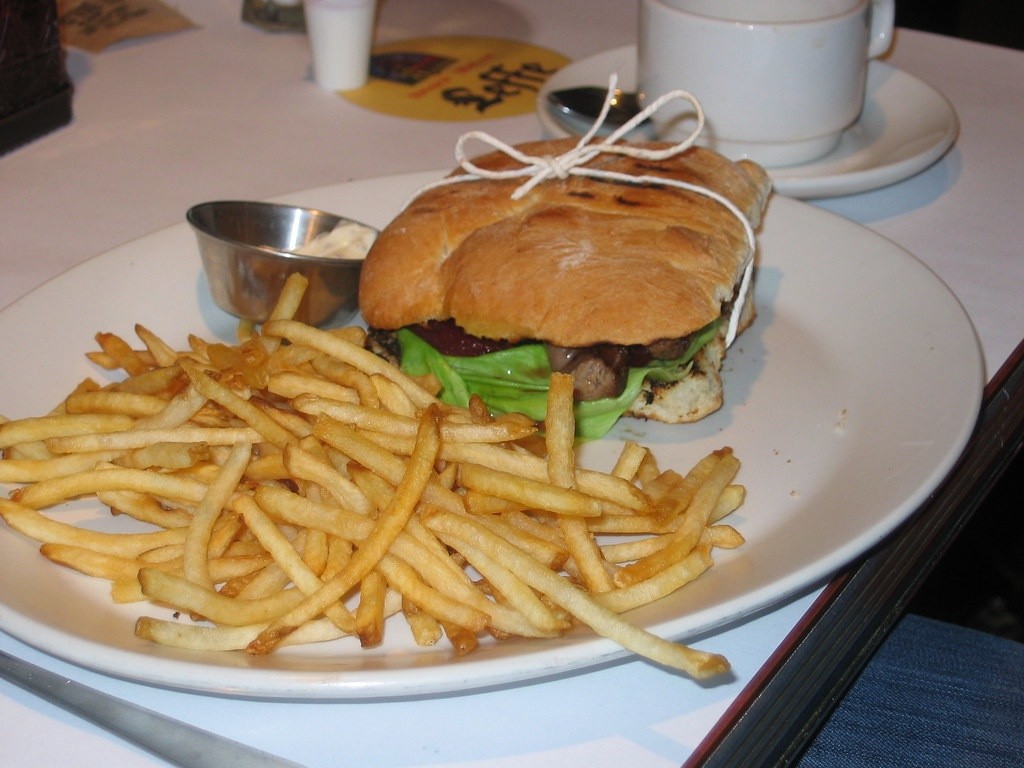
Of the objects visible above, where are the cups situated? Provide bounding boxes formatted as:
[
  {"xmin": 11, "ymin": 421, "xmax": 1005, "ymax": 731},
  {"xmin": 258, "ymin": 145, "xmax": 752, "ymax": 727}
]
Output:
[
  {"xmin": 302, "ymin": 0, "xmax": 377, "ymax": 90},
  {"xmin": 636, "ymin": 0, "xmax": 897, "ymax": 167}
]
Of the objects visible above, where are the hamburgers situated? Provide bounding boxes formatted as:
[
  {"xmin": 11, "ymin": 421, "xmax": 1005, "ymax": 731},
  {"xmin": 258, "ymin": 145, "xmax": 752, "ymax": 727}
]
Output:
[{"xmin": 360, "ymin": 132, "xmax": 774, "ymax": 450}]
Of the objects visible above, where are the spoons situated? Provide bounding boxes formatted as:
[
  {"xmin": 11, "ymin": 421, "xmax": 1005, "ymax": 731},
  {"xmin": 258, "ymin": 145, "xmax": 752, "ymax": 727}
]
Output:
[{"xmin": 544, "ymin": 87, "xmax": 647, "ymax": 137}]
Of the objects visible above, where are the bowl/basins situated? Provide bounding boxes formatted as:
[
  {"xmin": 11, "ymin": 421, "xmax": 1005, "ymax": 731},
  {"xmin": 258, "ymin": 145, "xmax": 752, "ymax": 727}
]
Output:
[{"xmin": 187, "ymin": 199, "xmax": 383, "ymax": 328}]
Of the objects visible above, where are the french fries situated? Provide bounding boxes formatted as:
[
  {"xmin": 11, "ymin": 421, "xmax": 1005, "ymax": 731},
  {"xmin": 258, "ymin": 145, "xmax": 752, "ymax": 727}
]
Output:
[{"xmin": 0, "ymin": 273, "xmax": 746, "ymax": 683}]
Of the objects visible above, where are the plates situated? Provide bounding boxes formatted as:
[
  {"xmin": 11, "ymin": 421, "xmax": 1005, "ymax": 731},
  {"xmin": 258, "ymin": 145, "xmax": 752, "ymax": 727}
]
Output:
[
  {"xmin": 0, "ymin": 160, "xmax": 987, "ymax": 711},
  {"xmin": 544, "ymin": 37, "xmax": 960, "ymax": 201}
]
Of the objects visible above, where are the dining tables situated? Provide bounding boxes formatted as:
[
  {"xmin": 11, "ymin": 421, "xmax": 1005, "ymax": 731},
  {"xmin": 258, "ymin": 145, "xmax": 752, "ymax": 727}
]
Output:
[{"xmin": 0, "ymin": 1, "xmax": 1024, "ymax": 768}]
